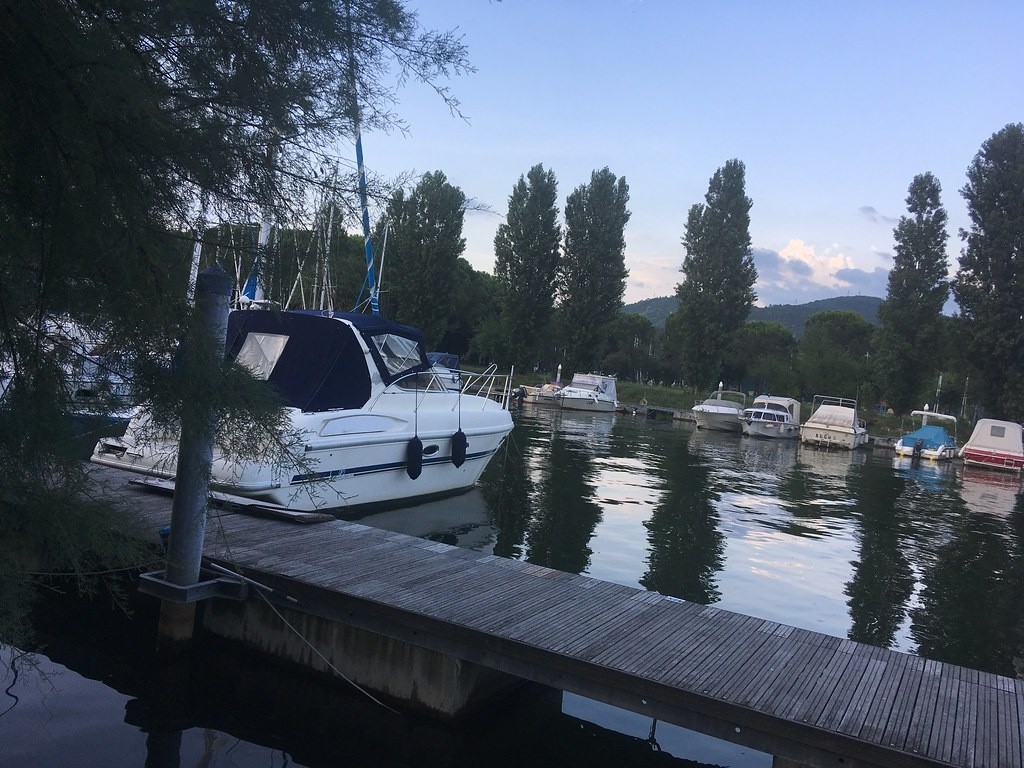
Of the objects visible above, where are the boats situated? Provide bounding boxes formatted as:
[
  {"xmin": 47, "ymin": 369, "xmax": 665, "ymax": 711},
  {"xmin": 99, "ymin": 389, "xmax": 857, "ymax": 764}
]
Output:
[
  {"xmin": 86, "ymin": 308, "xmax": 514, "ymax": 523},
  {"xmin": 691, "ymin": 381, "xmax": 748, "ymax": 433},
  {"xmin": 895, "ymin": 425, "xmax": 960, "ymax": 461},
  {"xmin": 0, "ymin": 311, "xmax": 168, "ymax": 413},
  {"xmin": 962, "ymin": 418, "xmax": 1024, "ymax": 473},
  {"xmin": 799, "ymin": 395, "xmax": 869, "ymax": 450},
  {"xmin": 520, "ymin": 383, "xmax": 563, "ymax": 404},
  {"xmin": 737, "ymin": 394, "xmax": 803, "ymax": 439},
  {"xmin": 555, "ymin": 373, "xmax": 626, "ymax": 413}
]
